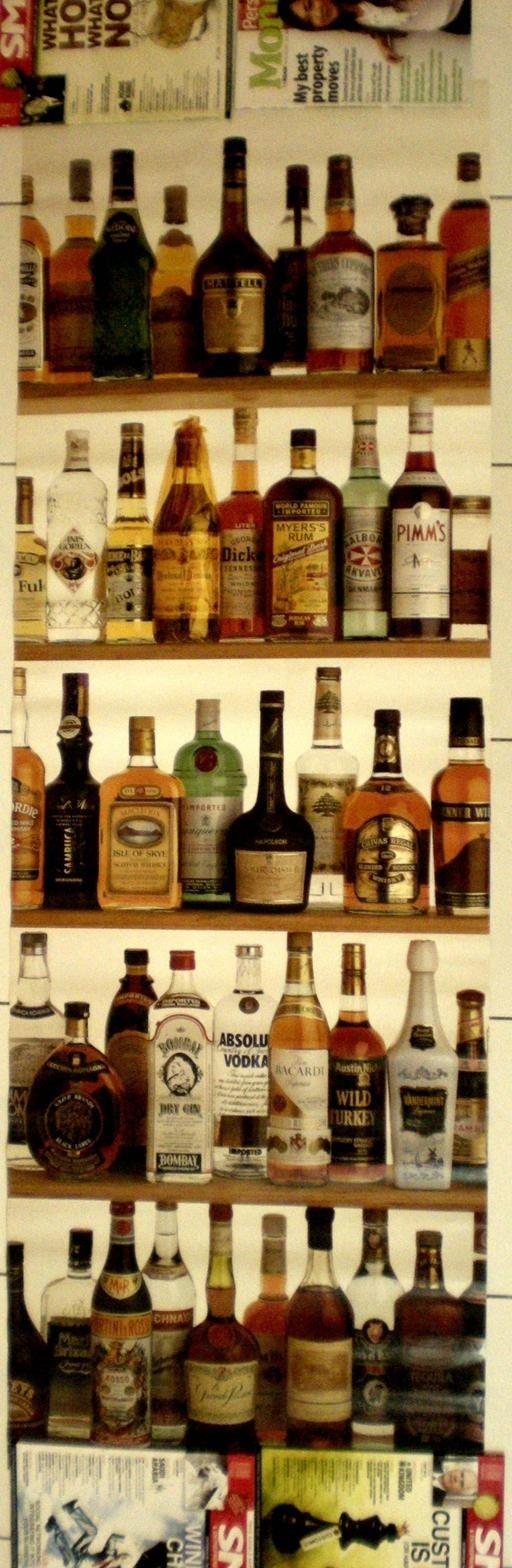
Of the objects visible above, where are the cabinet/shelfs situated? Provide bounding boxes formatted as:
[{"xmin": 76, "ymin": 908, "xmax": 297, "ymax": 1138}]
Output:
[{"xmin": 0, "ymin": 0, "xmax": 511, "ymax": 1568}]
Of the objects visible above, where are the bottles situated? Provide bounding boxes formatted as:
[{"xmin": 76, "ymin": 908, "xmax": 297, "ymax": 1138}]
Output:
[
  {"xmin": 103, "ymin": 421, "xmax": 154, "ymax": 643},
  {"xmin": 91, "ymin": 147, "xmax": 154, "ymax": 382},
  {"xmin": 15, "ymin": 474, "xmax": 49, "ymax": 643},
  {"xmin": 459, "ymin": 1210, "xmax": 485, "ymax": 1453},
  {"xmin": 106, "ymin": 946, "xmax": 156, "ymax": 1171},
  {"xmin": 332, "ymin": 402, "xmax": 392, "ymax": 640},
  {"xmin": 148, "ymin": 951, "xmax": 218, "ymax": 1187},
  {"xmin": 191, "ymin": 1383, "xmax": 199, "ymax": 1387},
  {"xmin": 217, "ymin": 407, "xmax": 264, "ymax": 646},
  {"xmin": 171, "ymin": 697, "xmax": 248, "ymax": 907},
  {"xmin": 432, "ymin": 694, "xmax": 491, "ymax": 919},
  {"xmin": 141, "ymin": 1200, "xmax": 196, "ymax": 1439},
  {"xmin": 388, "ymin": 938, "xmax": 456, "ymax": 1191},
  {"xmin": 266, "ymin": 426, "xmax": 343, "ymax": 641},
  {"xmin": 6, "ymin": 1239, "xmax": 50, "ymax": 1439},
  {"xmin": 267, "ymin": 929, "xmax": 331, "ymax": 1185},
  {"xmin": 98, "ymin": 713, "xmax": 184, "ymax": 912},
  {"xmin": 149, "ymin": 175, "xmax": 198, "ymax": 377},
  {"xmin": 396, "ymin": 1226, "xmax": 464, "ymax": 1446},
  {"xmin": 448, "ymin": 495, "xmax": 492, "ymax": 642},
  {"xmin": 37, "ymin": 1225, "xmax": 96, "ymax": 1440},
  {"xmin": 46, "ymin": 156, "xmax": 99, "ymax": 373},
  {"xmin": 347, "ymin": 1210, "xmax": 405, "ymax": 1438},
  {"xmin": 22, "ymin": 173, "xmax": 52, "ymax": 379},
  {"xmin": 305, "ymin": 152, "xmax": 374, "ymax": 379},
  {"xmin": 265, "ymin": 163, "xmax": 319, "ymax": 370},
  {"xmin": 153, "ymin": 415, "xmax": 219, "ymax": 641},
  {"xmin": 243, "ymin": 1214, "xmax": 291, "ymax": 1440},
  {"xmin": 285, "ymin": 1206, "xmax": 355, "ymax": 1444},
  {"xmin": 12, "ymin": 667, "xmax": 47, "ymax": 910},
  {"xmin": 9, "ymin": 930, "xmax": 67, "ymax": 1180},
  {"xmin": 24, "ymin": 999, "xmax": 131, "ymax": 1178},
  {"xmin": 340, "ymin": 708, "xmax": 432, "ymax": 917},
  {"xmin": 45, "ymin": 670, "xmax": 101, "ymax": 909},
  {"xmin": 189, "ymin": 1199, "xmax": 258, "ymax": 1449},
  {"xmin": 212, "ymin": 941, "xmax": 275, "ymax": 1181},
  {"xmin": 450, "ymin": 990, "xmax": 487, "ymax": 1188},
  {"xmin": 88, "ymin": 1199, "xmax": 152, "ymax": 1448},
  {"xmin": 439, "ymin": 150, "xmax": 490, "ymax": 376},
  {"xmin": 387, "ymin": 395, "xmax": 453, "ymax": 642},
  {"xmin": 43, "ymin": 427, "xmax": 106, "ymax": 645},
  {"xmin": 296, "ymin": 665, "xmax": 359, "ymax": 907},
  {"xmin": 195, "ymin": 133, "xmax": 270, "ymax": 376},
  {"xmin": 376, "ymin": 193, "xmax": 446, "ymax": 372},
  {"xmin": 328, "ymin": 939, "xmax": 386, "ymax": 1183},
  {"xmin": 228, "ymin": 688, "xmax": 315, "ymax": 913}
]
